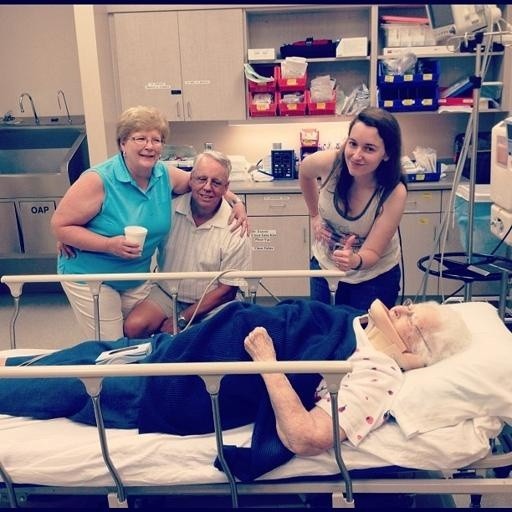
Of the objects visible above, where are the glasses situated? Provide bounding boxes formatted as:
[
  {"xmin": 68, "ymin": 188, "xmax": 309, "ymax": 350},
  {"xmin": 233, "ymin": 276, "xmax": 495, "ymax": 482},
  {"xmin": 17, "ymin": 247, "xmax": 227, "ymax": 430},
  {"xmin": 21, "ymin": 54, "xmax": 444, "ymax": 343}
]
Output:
[
  {"xmin": 129, "ymin": 136, "xmax": 164, "ymax": 146},
  {"xmin": 403, "ymin": 297, "xmax": 431, "ymax": 353}
]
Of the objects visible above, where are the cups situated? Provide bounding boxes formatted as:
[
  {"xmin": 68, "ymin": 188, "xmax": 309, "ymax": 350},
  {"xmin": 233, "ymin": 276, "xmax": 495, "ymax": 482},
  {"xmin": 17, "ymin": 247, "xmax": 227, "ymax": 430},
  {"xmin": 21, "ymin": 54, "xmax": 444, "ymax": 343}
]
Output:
[{"xmin": 123, "ymin": 224, "xmax": 148, "ymax": 257}]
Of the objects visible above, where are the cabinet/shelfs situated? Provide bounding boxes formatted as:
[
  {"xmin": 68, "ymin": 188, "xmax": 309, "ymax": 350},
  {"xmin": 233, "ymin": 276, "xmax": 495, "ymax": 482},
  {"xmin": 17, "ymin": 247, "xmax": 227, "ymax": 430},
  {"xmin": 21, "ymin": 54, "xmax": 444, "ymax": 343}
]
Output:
[
  {"xmin": 112, "ymin": 9, "xmax": 246, "ymax": 122},
  {"xmin": 237, "ymin": 190, "xmax": 309, "ymax": 295},
  {"xmin": 398, "ymin": 191, "xmax": 508, "ymax": 295},
  {"xmin": 242, "ymin": 4, "xmax": 512, "ymax": 123}
]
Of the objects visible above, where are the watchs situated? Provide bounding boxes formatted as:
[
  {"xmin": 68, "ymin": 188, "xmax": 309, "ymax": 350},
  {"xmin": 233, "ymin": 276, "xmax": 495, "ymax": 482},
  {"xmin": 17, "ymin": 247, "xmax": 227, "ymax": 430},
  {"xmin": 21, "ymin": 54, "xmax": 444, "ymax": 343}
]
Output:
[{"xmin": 176, "ymin": 312, "xmax": 190, "ymax": 330}]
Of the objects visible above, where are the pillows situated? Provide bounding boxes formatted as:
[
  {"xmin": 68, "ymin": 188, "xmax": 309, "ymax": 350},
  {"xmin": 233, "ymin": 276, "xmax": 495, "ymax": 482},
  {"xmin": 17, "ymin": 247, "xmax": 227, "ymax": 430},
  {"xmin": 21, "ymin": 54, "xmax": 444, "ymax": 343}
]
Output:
[{"xmin": 390, "ymin": 301, "xmax": 512, "ymax": 439}]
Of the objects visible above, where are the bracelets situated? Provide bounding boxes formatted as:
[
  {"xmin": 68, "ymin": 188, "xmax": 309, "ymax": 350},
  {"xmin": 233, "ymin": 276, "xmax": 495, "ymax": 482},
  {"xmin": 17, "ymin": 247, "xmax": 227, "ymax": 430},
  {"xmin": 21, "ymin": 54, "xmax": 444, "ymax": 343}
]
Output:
[
  {"xmin": 230, "ymin": 195, "xmax": 248, "ymax": 212},
  {"xmin": 351, "ymin": 253, "xmax": 363, "ymax": 271}
]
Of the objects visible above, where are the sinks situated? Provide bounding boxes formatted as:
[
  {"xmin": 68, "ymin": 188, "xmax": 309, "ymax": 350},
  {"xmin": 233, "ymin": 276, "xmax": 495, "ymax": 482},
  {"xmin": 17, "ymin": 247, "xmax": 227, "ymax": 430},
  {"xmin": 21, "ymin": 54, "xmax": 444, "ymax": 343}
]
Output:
[{"xmin": 0, "ymin": 126, "xmax": 87, "ymax": 177}]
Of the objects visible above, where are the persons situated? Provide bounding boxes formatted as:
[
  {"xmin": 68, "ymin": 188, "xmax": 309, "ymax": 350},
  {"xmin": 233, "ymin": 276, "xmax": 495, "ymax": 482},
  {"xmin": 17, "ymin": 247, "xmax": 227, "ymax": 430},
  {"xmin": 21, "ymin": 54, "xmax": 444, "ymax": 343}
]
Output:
[
  {"xmin": 0, "ymin": 298, "xmax": 475, "ymax": 483},
  {"xmin": 298, "ymin": 108, "xmax": 407, "ymax": 311},
  {"xmin": 49, "ymin": 107, "xmax": 251, "ymax": 344},
  {"xmin": 54, "ymin": 148, "xmax": 253, "ymax": 344}
]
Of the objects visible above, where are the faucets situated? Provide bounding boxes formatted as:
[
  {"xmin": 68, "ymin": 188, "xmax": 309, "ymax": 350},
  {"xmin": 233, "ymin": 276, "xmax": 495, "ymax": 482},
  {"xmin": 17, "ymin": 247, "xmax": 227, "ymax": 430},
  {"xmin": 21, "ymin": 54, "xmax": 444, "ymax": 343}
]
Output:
[
  {"xmin": 19, "ymin": 93, "xmax": 40, "ymax": 124},
  {"xmin": 56, "ymin": 90, "xmax": 72, "ymax": 123}
]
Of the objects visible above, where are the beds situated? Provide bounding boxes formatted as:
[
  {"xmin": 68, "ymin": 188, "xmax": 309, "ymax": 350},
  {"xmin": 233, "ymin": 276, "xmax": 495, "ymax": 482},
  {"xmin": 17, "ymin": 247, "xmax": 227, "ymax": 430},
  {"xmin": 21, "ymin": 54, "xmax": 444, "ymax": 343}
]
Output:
[{"xmin": 1, "ymin": 269, "xmax": 512, "ymax": 511}]
